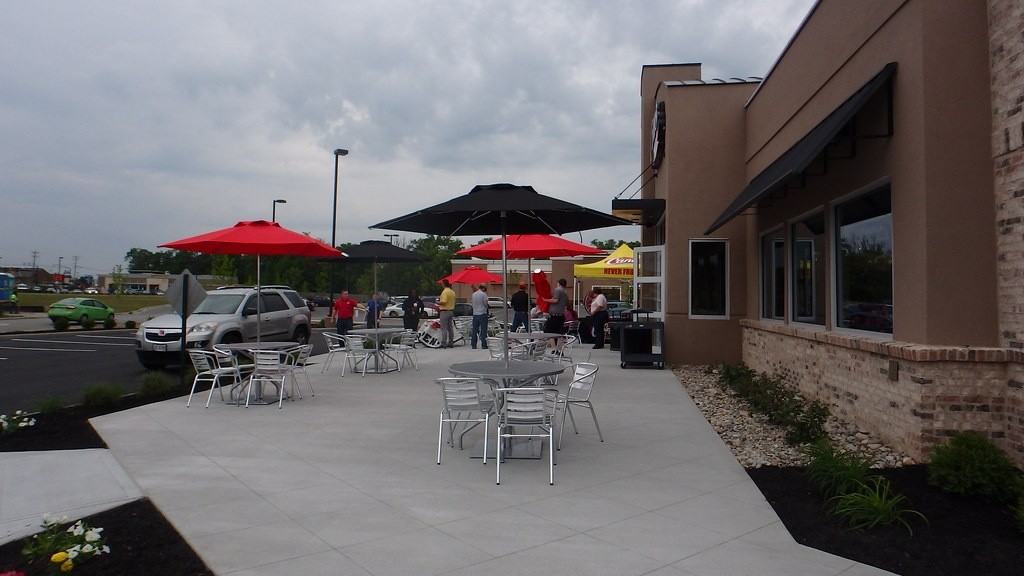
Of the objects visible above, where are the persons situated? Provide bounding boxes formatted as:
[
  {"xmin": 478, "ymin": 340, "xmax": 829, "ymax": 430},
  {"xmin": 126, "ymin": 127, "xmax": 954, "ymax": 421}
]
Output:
[
  {"xmin": 402, "ymin": 288, "xmax": 425, "ymax": 349},
  {"xmin": 508, "ymin": 281, "xmax": 535, "ymax": 350},
  {"xmin": 591, "ymin": 288, "xmax": 608, "ymax": 349},
  {"xmin": 471, "ymin": 283, "xmax": 488, "ymax": 349},
  {"xmin": 365, "ymin": 290, "xmax": 384, "ymax": 329},
  {"xmin": 434, "ymin": 280, "xmax": 456, "ymax": 348},
  {"xmin": 331, "ymin": 289, "xmax": 370, "ymax": 347},
  {"xmin": 562, "ymin": 302, "xmax": 577, "ymax": 337},
  {"xmin": 541, "ymin": 278, "xmax": 568, "ymax": 358}
]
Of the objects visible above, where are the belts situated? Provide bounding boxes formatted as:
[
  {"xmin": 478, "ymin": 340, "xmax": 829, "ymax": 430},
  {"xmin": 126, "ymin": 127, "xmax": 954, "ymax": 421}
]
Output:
[{"xmin": 551, "ymin": 314, "xmax": 564, "ymax": 317}]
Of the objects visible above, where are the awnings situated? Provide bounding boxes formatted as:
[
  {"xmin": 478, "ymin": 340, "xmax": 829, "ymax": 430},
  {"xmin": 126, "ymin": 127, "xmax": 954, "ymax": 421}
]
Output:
[{"xmin": 703, "ymin": 67, "xmax": 892, "ymax": 237}]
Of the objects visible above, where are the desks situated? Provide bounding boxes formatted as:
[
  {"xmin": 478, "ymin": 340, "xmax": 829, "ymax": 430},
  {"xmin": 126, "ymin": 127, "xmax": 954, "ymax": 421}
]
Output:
[
  {"xmin": 347, "ymin": 328, "xmax": 405, "ymax": 374},
  {"xmin": 497, "ymin": 332, "xmax": 561, "ymax": 380},
  {"xmin": 448, "ymin": 357, "xmax": 564, "ymax": 460},
  {"xmin": 214, "ymin": 341, "xmax": 300, "ymax": 405}
]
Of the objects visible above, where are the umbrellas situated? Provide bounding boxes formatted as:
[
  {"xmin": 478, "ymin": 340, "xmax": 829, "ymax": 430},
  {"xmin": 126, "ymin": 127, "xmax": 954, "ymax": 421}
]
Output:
[
  {"xmin": 368, "ymin": 183, "xmax": 636, "ymax": 372},
  {"xmin": 316, "ymin": 240, "xmax": 435, "ymax": 350},
  {"xmin": 157, "ymin": 220, "xmax": 349, "ymax": 345}
]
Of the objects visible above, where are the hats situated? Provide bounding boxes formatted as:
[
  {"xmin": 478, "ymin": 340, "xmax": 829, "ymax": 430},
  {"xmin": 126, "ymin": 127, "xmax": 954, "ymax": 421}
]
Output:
[
  {"xmin": 520, "ymin": 282, "xmax": 528, "ymax": 287},
  {"xmin": 557, "ymin": 279, "xmax": 567, "ymax": 285},
  {"xmin": 479, "ymin": 283, "xmax": 487, "ymax": 291}
]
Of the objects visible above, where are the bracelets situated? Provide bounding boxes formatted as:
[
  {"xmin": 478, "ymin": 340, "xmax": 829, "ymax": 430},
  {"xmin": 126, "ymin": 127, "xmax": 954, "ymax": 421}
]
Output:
[{"xmin": 378, "ymin": 318, "xmax": 381, "ymax": 320}]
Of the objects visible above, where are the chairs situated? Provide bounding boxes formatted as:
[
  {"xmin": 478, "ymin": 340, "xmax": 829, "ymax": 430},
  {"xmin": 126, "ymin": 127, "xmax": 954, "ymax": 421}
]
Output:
[{"xmin": 185, "ymin": 314, "xmax": 612, "ymax": 485}]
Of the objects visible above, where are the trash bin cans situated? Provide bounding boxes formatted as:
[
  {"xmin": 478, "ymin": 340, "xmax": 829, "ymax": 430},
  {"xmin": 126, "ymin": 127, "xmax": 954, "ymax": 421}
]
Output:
[
  {"xmin": 608, "ymin": 307, "xmax": 633, "ymax": 351},
  {"xmin": 619, "ymin": 309, "xmax": 654, "ymax": 366}
]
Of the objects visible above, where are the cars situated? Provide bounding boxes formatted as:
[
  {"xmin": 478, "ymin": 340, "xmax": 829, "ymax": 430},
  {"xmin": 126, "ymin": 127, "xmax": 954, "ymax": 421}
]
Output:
[
  {"xmin": 303, "ymin": 294, "xmax": 511, "ymax": 318},
  {"xmin": 48, "ymin": 298, "xmax": 115, "ymax": 323},
  {"xmin": 16, "ymin": 284, "xmax": 165, "ymax": 295}
]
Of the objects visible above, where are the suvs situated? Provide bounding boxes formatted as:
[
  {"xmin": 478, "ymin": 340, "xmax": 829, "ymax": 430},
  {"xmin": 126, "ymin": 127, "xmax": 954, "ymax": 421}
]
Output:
[{"xmin": 135, "ymin": 284, "xmax": 311, "ymax": 366}]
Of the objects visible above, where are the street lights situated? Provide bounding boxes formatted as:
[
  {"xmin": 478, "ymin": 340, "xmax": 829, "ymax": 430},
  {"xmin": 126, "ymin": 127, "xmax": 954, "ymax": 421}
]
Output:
[
  {"xmin": 59, "ymin": 257, "xmax": 63, "ymax": 275},
  {"xmin": 272, "ymin": 199, "xmax": 287, "ymax": 222},
  {"xmin": 329, "ymin": 149, "xmax": 349, "ymax": 318}
]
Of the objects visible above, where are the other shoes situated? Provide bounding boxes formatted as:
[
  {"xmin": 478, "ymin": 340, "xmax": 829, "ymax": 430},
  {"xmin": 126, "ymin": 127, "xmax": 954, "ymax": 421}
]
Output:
[
  {"xmin": 593, "ymin": 345, "xmax": 604, "ymax": 349},
  {"xmin": 472, "ymin": 346, "xmax": 477, "ymax": 349},
  {"xmin": 482, "ymin": 346, "xmax": 488, "ymax": 349},
  {"xmin": 551, "ymin": 350, "xmax": 564, "ymax": 356}
]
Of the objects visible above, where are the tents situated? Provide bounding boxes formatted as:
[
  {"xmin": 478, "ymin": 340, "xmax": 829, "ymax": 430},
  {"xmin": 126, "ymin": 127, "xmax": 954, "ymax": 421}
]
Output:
[{"xmin": 573, "ymin": 243, "xmax": 641, "ymax": 318}]
